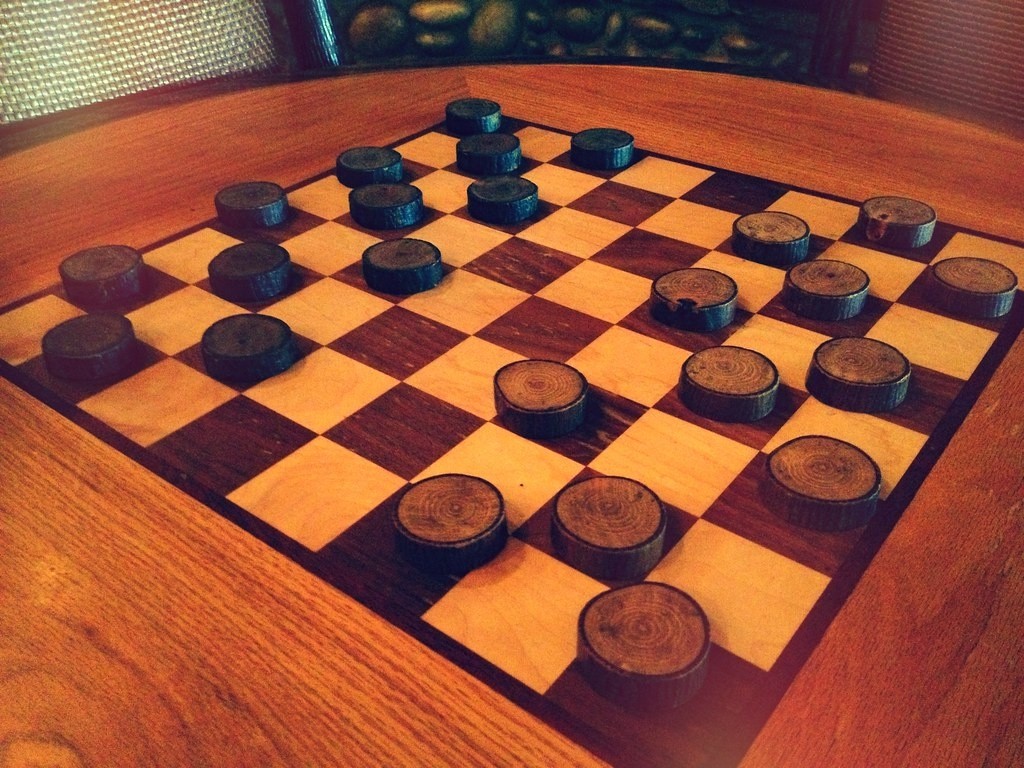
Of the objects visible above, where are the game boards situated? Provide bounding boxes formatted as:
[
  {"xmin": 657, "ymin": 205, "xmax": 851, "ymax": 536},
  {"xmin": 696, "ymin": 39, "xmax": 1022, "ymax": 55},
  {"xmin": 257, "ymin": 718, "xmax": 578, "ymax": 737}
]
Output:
[{"xmin": 0, "ymin": 114, "xmax": 1024, "ymax": 768}]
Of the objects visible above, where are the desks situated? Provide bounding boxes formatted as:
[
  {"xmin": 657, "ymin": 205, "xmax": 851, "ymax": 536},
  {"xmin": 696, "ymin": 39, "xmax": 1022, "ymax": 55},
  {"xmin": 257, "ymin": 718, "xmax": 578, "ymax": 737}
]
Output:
[{"xmin": 0, "ymin": 56, "xmax": 1024, "ymax": 766}]
